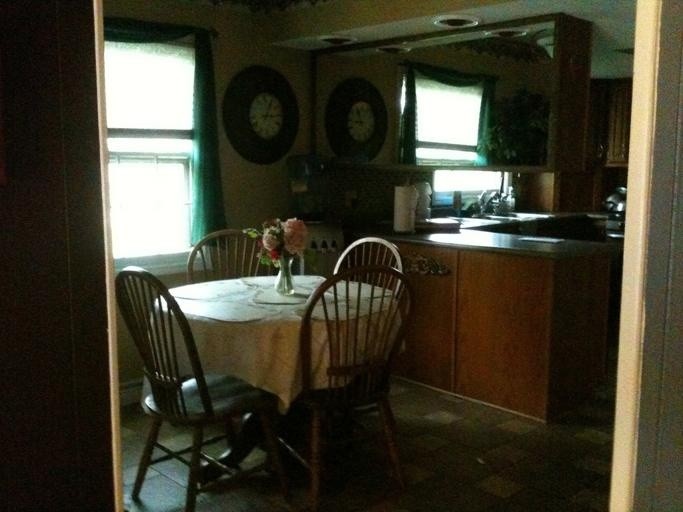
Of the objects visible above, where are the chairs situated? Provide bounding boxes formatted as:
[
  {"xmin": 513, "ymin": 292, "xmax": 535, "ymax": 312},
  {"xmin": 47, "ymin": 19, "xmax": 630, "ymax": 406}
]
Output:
[
  {"xmin": 114, "ymin": 265, "xmax": 291, "ymax": 511},
  {"xmin": 186, "ymin": 229, "xmax": 276, "ymax": 283},
  {"xmin": 265, "ymin": 264, "xmax": 416, "ymax": 511},
  {"xmin": 333, "ymin": 237, "xmax": 404, "ymax": 294}
]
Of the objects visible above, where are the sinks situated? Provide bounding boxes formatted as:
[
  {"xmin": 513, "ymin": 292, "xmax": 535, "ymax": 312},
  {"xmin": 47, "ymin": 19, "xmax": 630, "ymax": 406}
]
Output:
[
  {"xmin": 474, "ymin": 213, "xmax": 520, "ymax": 224},
  {"xmin": 490, "ymin": 211, "xmax": 555, "ymax": 219}
]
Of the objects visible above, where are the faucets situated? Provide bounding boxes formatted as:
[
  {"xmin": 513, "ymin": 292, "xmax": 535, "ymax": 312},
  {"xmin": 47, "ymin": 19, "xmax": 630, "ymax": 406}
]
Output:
[{"xmin": 480, "ymin": 191, "xmax": 501, "ymax": 212}]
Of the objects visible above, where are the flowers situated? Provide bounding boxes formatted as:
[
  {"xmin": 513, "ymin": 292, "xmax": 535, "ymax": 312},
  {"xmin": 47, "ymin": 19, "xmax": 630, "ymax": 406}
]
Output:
[{"xmin": 241, "ymin": 217, "xmax": 308, "ymax": 289}]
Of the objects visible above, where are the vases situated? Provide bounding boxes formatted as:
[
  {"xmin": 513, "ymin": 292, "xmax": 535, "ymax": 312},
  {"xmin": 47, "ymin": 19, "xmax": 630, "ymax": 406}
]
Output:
[{"xmin": 274, "ymin": 257, "xmax": 296, "ymax": 295}]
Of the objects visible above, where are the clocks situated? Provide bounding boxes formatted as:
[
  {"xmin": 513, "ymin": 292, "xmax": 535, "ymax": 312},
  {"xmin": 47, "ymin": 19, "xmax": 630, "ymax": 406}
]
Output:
[
  {"xmin": 222, "ymin": 66, "xmax": 301, "ymax": 164},
  {"xmin": 324, "ymin": 78, "xmax": 388, "ymax": 163}
]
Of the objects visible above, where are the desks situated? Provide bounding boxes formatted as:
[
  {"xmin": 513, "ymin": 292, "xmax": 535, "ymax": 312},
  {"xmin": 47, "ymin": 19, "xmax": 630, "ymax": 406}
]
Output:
[{"xmin": 139, "ymin": 275, "xmax": 405, "ymax": 485}]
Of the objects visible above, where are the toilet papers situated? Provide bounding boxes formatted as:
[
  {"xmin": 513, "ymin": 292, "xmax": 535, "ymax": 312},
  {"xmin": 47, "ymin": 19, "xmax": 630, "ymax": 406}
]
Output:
[{"xmin": 393, "ymin": 185, "xmax": 417, "ymax": 233}]
